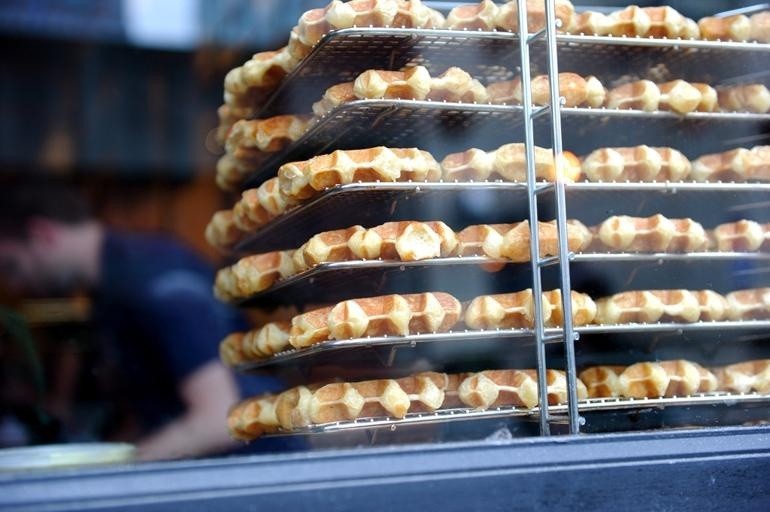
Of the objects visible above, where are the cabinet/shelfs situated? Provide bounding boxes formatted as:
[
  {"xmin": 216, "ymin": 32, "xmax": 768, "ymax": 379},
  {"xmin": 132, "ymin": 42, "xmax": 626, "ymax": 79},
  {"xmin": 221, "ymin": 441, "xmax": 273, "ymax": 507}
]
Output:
[{"xmin": 221, "ymin": 23, "xmax": 769, "ymax": 440}]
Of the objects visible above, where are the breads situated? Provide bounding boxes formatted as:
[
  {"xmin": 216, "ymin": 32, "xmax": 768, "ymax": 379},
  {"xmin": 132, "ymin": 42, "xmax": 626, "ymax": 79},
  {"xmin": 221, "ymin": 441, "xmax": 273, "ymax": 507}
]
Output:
[{"xmin": 202, "ymin": 1, "xmax": 770, "ymax": 440}]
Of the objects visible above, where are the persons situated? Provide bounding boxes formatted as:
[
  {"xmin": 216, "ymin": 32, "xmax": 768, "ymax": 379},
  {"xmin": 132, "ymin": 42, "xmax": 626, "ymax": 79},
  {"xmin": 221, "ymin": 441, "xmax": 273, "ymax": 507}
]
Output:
[{"xmin": 0, "ymin": 179, "xmax": 313, "ymax": 459}]
